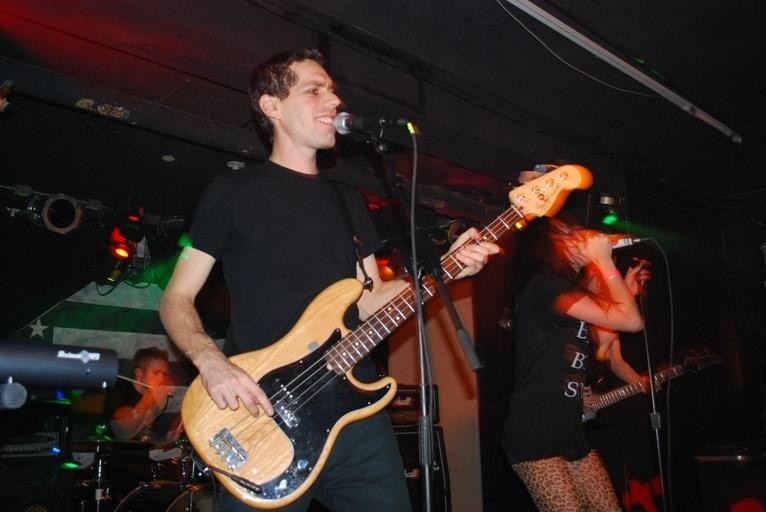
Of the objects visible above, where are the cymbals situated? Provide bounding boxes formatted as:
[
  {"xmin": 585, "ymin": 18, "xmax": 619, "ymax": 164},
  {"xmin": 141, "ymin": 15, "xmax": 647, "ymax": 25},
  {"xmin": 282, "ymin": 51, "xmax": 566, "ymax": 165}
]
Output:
[{"xmin": 66, "ymin": 436, "xmax": 152, "ymax": 452}]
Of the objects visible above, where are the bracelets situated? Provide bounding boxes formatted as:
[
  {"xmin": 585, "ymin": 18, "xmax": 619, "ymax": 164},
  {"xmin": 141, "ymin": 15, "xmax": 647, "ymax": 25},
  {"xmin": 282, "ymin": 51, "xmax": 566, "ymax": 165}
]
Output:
[{"xmin": 600, "ymin": 270, "xmax": 622, "ymax": 282}]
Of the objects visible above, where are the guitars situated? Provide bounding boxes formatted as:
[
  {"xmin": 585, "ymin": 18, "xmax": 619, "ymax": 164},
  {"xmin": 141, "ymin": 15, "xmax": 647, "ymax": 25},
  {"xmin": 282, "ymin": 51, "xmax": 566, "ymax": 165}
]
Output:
[
  {"xmin": 181, "ymin": 163, "xmax": 594, "ymax": 509},
  {"xmin": 582, "ymin": 357, "xmax": 702, "ymax": 422}
]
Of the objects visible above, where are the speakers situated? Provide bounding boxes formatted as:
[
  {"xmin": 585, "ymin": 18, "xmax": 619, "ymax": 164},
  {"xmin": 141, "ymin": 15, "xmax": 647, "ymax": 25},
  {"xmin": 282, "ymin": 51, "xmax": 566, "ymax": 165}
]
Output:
[{"xmin": 390, "ymin": 425, "xmax": 451, "ymax": 512}]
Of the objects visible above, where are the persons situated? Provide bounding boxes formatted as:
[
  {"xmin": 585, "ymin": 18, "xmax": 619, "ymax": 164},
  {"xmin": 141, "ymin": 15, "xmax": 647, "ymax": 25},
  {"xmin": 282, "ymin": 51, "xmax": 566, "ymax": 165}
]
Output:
[
  {"xmin": 579, "ymin": 242, "xmax": 661, "ymax": 512},
  {"xmin": 499, "ymin": 206, "xmax": 657, "ymax": 511},
  {"xmin": 157, "ymin": 44, "xmax": 504, "ymax": 511},
  {"xmin": 101, "ymin": 345, "xmax": 183, "ymax": 511}
]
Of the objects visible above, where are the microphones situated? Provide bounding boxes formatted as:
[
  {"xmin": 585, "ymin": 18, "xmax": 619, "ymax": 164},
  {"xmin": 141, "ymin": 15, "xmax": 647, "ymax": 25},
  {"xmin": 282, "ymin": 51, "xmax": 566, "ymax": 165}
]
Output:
[
  {"xmin": 611, "ymin": 237, "xmax": 641, "ymax": 249},
  {"xmin": 334, "ymin": 112, "xmax": 408, "ymax": 135}
]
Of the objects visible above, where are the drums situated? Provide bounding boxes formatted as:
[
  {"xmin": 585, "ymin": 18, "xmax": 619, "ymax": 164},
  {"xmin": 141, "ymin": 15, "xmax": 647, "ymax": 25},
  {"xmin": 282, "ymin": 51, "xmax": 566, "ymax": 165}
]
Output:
[
  {"xmin": 117, "ymin": 481, "xmax": 213, "ymax": 511},
  {"xmin": 150, "ymin": 441, "xmax": 213, "ymax": 484}
]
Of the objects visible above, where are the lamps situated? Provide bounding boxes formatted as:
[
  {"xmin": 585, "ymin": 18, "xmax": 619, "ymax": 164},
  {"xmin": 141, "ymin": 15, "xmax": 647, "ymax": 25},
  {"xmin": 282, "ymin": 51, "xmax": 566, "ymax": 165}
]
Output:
[{"xmin": 107, "ymin": 206, "xmax": 145, "ymax": 283}]
